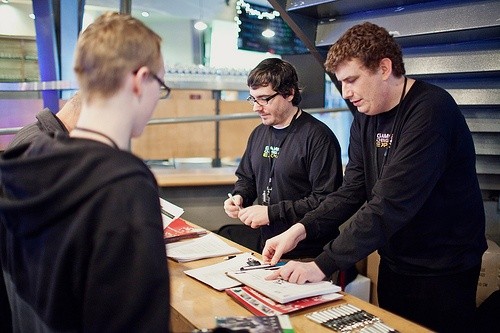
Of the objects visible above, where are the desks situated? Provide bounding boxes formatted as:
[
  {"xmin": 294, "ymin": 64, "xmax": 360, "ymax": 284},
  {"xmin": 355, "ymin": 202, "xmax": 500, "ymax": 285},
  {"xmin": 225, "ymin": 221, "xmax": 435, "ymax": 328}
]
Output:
[{"xmin": 167, "ymin": 221, "xmax": 438, "ymax": 333}]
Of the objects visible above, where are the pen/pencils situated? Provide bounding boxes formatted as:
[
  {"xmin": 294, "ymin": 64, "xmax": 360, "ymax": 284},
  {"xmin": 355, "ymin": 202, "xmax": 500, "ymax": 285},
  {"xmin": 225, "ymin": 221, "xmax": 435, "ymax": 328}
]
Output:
[
  {"xmin": 240, "ymin": 264, "xmax": 270, "ymax": 270},
  {"xmin": 228, "ymin": 193, "xmax": 241, "ymax": 211}
]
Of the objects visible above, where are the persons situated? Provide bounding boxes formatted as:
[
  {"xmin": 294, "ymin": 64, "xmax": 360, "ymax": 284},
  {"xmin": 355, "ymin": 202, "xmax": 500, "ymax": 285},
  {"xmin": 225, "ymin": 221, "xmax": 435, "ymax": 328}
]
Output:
[
  {"xmin": 263, "ymin": 22, "xmax": 489, "ymax": 333},
  {"xmin": 6, "ymin": 93, "xmax": 83, "ymax": 152},
  {"xmin": 216, "ymin": 58, "xmax": 345, "ymax": 258},
  {"xmin": 0, "ymin": 12, "xmax": 171, "ymax": 333}
]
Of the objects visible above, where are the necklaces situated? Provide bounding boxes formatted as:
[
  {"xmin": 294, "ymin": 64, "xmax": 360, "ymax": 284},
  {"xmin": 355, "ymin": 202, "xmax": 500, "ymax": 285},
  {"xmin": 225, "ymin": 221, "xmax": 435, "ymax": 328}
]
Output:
[{"xmin": 75, "ymin": 127, "xmax": 118, "ymax": 149}]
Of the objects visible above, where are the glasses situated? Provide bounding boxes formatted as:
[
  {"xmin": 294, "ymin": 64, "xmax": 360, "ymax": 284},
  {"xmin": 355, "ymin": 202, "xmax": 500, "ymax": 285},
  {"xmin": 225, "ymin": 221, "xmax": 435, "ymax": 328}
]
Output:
[
  {"xmin": 247, "ymin": 92, "xmax": 280, "ymax": 106},
  {"xmin": 132, "ymin": 67, "xmax": 172, "ymax": 100}
]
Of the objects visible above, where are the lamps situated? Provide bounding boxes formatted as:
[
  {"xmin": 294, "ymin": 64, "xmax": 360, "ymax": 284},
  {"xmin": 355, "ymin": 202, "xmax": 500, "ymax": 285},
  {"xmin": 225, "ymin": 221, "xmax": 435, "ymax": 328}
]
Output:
[{"xmin": 261, "ymin": 21, "xmax": 276, "ymax": 39}]
